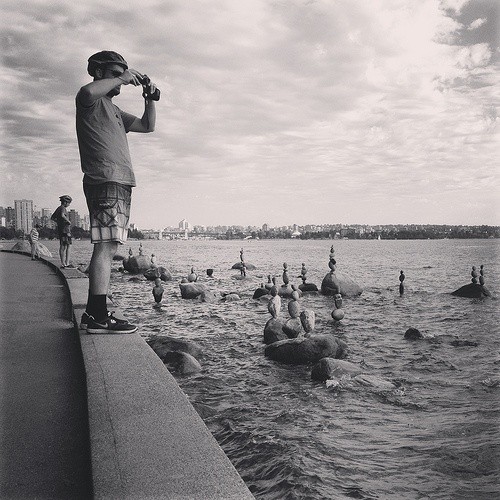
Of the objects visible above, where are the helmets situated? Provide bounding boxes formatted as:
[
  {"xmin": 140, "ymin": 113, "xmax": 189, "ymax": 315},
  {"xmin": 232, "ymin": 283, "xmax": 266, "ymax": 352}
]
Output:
[
  {"xmin": 59, "ymin": 195, "xmax": 72, "ymax": 202},
  {"xmin": 87, "ymin": 51, "xmax": 128, "ymax": 76}
]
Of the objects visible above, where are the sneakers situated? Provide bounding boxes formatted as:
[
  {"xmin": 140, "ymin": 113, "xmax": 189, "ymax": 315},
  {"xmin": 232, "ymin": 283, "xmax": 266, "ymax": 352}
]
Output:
[
  {"xmin": 61, "ymin": 264, "xmax": 64, "ymax": 268},
  {"xmin": 64, "ymin": 264, "xmax": 78, "ymax": 270},
  {"xmin": 80, "ymin": 311, "xmax": 128, "ymax": 330},
  {"xmin": 86, "ymin": 316, "xmax": 138, "ymax": 334}
]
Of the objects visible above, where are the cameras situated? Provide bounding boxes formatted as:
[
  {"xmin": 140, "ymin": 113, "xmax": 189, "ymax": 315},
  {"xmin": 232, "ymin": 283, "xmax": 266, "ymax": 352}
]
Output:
[{"xmin": 140, "ymin": 75, "xmax": 160, "ymax": 100}]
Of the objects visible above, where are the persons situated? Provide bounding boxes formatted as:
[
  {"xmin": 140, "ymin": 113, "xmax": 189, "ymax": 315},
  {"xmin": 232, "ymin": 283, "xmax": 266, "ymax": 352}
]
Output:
[
  {"xmin": 75, "ymin": 51, "xmax": 155, "ymax": 334},
  {"xmin": 28, "ymin": 224, "xmax": 42, "ymax": 261},
  {"xmin": 51, "ymin": 195, "xmax": 77, "ymax": 269}
]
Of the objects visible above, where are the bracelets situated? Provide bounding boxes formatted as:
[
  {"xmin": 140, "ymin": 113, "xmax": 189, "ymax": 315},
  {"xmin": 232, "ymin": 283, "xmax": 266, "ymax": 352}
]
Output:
[{"xmin": 118, "ymin": 76, "xmax": 129, "ymax": 83}]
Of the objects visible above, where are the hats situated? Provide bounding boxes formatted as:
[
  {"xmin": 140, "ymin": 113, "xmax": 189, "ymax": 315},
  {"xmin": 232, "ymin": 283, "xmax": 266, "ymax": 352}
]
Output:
[{"xmin": 34, "ymin": 224, "xmax": 41, "ymax": 228}]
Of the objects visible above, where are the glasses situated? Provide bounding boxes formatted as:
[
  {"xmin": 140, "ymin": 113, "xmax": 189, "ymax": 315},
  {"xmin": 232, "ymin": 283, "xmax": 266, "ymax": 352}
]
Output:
[{"xmin": 103, "ymin": 69, "xmax": 122, "ymax": 77}]
